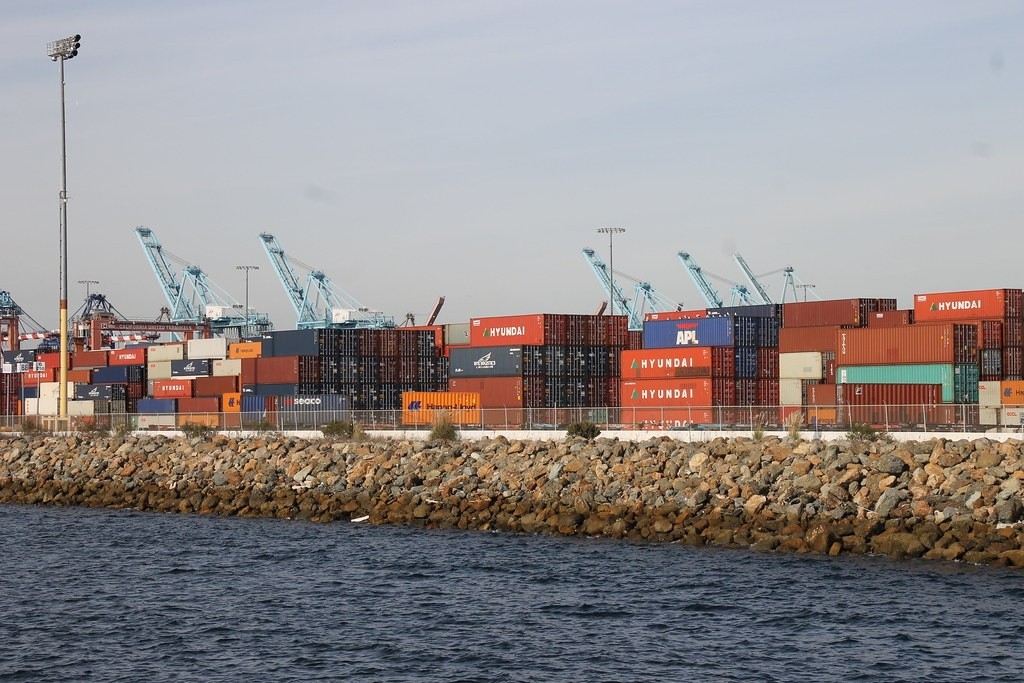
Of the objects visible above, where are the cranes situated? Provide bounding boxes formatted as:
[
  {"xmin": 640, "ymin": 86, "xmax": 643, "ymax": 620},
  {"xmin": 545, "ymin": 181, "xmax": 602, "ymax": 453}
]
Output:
[
  {"xmin": 133, "ymin": 224, "xmax": 401, "ymax": 343},
  {"xmin": 580, "ymin": 247, "xmax": 818, "ymax": 332}
]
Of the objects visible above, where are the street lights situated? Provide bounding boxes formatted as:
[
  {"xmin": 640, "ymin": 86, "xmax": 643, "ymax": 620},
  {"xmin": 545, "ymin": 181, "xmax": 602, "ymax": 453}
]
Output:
[
  {"xmin": 597, "ymin": 227, "xmax": 626, "ymax": 314},
  {"xmin": 45, "ymin": 33, "xmax": 81, "ymax": 437}
]
